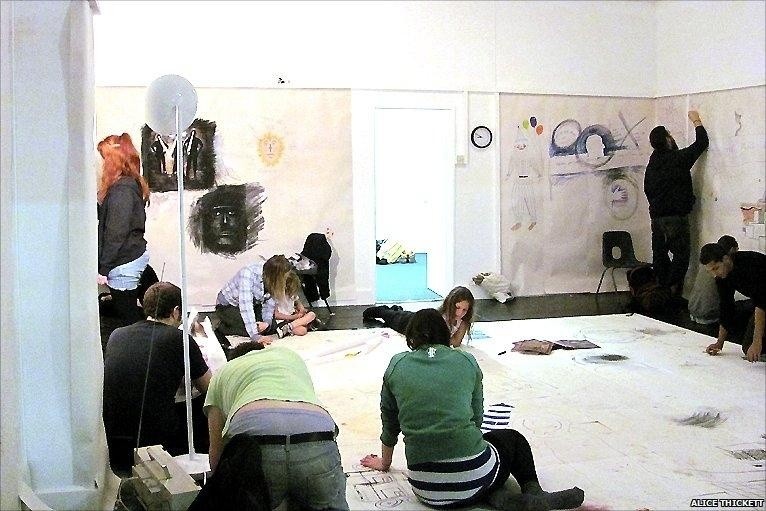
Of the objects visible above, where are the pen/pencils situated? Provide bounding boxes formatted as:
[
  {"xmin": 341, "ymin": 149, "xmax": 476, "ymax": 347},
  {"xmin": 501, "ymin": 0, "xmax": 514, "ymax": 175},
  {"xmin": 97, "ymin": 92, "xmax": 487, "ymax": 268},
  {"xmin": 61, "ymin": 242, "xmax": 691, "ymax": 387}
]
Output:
[{"xmin": 712, "ymin": 349, "xmax": 719, "ymax": 351}]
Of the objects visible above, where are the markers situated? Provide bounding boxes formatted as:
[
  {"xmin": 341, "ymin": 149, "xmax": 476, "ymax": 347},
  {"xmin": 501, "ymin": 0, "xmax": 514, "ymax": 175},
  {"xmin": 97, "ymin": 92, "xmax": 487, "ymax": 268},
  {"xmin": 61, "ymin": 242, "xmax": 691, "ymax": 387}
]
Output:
[{"xmin": 498, "ymin": 351, "xmax": 506, "ymax": 356}]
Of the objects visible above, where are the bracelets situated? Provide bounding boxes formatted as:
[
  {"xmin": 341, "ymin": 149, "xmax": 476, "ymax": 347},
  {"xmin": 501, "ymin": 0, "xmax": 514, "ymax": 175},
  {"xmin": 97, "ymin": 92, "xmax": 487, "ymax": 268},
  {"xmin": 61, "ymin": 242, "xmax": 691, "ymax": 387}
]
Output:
[{"xmin": 691, "ymin": 119, "xmax": 701, "ymax": 125}]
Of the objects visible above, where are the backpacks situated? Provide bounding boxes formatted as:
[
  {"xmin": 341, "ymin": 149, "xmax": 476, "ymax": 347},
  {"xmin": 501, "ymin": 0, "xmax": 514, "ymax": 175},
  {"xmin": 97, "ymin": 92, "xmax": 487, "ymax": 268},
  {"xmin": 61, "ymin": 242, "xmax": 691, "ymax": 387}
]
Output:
[{"xmin": 627, "ymin": 266, "xmax": 672, "ymax": 316}]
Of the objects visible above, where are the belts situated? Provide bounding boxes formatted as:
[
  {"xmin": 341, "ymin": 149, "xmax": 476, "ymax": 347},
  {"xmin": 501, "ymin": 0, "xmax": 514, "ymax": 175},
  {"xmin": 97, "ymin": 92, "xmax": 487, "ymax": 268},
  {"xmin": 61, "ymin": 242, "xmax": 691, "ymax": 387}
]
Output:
[{"xmin": 251, "ymin": 431, "xmax": 332, "ymax": 445}]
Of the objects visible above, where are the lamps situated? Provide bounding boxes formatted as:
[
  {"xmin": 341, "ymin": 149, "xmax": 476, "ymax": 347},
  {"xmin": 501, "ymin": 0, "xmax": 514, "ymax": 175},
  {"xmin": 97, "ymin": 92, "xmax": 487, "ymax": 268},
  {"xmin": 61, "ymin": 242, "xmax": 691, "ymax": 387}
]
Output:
[{"xmin": 141, "ymin": 73, "xmax": 230, "ymax": 481}]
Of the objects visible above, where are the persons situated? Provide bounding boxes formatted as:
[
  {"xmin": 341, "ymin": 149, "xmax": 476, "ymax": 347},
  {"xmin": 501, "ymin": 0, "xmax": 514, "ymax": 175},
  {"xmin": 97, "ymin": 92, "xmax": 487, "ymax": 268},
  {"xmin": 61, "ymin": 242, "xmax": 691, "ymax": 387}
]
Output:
[
  {"xmin": 645, "ymin": 105, "xmax": 710, "ymax": 321},
  {"xmin": 273, "ymin": 270, "xmax": 316, "ymax": 339},
  {"xmin": 98, "ymin": 133, "xmax": 151, "ymax": 325},
  {"xmin": 687, "ymin": 233, "xmax": 739, "ymax": 332},
  {"xmin": 361, "ymin": 285, "xmax": 475, "ymax": 349},
  {"xmin": 186, "ymin": 338, "xmax": 350, "ymax": 511},
  {"xmin": 214, "ymin": 255, "xmax": 292, "ymax": 347},
  {"xmin": 359, "ymin": 307, "xmax": 584, "ymax": 511},
  {"xmin": 700, "ymin": 244, "xmax": 765, "ymax": 363},
  {"xmin": 103, "ymin": 281, "xmax": 214, "ymax": 479}
]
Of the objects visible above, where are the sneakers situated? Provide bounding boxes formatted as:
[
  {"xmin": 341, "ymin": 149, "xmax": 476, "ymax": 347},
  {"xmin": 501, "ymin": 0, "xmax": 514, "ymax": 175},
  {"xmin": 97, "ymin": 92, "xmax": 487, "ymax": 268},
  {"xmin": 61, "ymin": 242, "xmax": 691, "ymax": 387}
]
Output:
[
  {"xmin": 276, "ymin": 323, "xmax": 293, "ymax": 339},
  {"xmin": 363, "ymin": 305, "xmax": 403, "ymax": 318},
  {"xmin": 310, "ymin": 319, "xmax": 321, "ymax": 331}
]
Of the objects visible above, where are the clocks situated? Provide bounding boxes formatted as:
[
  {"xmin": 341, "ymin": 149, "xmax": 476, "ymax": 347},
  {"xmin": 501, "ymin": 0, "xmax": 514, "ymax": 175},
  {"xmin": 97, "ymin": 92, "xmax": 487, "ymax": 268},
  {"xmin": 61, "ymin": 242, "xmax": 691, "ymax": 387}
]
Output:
[{"xmin": 470, "ymin": 123, "xmax": 494, "ymax": 149}]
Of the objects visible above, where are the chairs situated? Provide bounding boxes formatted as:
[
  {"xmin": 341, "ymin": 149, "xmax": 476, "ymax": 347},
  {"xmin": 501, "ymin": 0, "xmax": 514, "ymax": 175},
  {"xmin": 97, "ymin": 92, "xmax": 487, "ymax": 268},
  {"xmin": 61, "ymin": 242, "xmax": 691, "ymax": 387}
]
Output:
[
  {"xmin": 290, "ymin": 232, "xmax": 336, "ymax": 326},
  {"xmin": 596, "ymin": 228, "xmax": 655, "ymax": 307}
]
